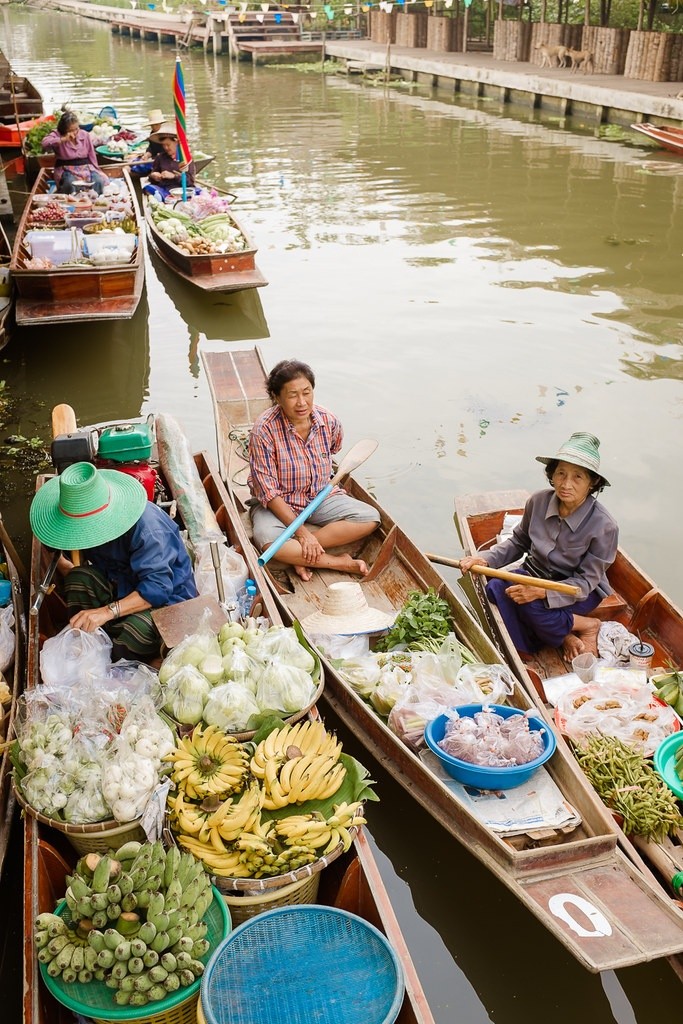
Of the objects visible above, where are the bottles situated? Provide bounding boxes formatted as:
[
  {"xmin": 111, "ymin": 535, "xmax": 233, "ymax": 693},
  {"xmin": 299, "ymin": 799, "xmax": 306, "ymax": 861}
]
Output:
[
  {"xmin": 236, "ymin": 579, "xmax": 255, "ymax": 600},
  {"xmin": 239, "ymin": 586, "xmax": 258, "ymax": 615}
]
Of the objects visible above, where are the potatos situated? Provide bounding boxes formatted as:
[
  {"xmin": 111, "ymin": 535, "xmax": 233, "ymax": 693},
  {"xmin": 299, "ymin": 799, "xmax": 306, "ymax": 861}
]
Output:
[{"xmin": 170, "ymin": 232, "xmax": 236, "ymax": 255}]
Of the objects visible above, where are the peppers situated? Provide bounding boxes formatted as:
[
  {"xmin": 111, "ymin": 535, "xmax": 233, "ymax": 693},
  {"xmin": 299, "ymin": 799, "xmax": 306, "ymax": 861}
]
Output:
[{"xmin": 70, "ymin": 703, "xmax": 128, "ymax": 740}]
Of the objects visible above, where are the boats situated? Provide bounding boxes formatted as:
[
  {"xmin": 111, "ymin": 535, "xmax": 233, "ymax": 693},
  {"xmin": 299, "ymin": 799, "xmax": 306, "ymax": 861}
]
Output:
[
  {"xmin": 453, "ymin": 489, "xmax": 683, "ymax": 909},
  {"xmin": 0, "ymin": 50, "xmax": 219, "ymax": 192},
  {"xmin": 20, "ymin": 403, "xmax": 435, "ymax": 1024},
  {"xmin": 9, "ymin": 163, "xmax": 144, "ymax": 328},
  {"xmin": 199, "ymin": 346, "xmax": 683, "ymax": 975},
  {"xmin": 629, "ymin": 123, "xmax": 683, "ymax": 156},
  {"xmin": 139, "ymin": 176, "xmax": 270, "ymax": 297}
]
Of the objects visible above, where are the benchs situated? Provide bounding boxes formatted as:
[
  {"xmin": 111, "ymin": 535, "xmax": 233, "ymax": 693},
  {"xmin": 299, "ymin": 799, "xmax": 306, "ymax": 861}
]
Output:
[{"xmin": 589, "ymin": 589, "xmax": 623, "ymax": 615}]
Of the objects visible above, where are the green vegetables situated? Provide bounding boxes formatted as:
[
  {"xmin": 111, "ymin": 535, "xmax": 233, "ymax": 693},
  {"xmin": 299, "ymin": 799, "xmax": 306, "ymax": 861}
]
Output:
[
  {"xmin": 373, "ymin": 586, "xmax": 479, "ymax": 671},
  {"xmin": 154, "ymin": 209, "xmax": 194, "ymax": 223},
  {"xmin": 184, "ymin": 221, "xmax": 205, "ymax": 236},
  {"xmin": 25, "ymin": 111, "xmax": 64, "ymax": 154}
]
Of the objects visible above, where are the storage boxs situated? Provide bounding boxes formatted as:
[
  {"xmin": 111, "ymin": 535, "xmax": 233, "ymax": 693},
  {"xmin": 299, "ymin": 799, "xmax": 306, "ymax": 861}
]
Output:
[
  {"xmin": 97, "ymin": 423, "xmax": 152, "ymax": 462},
  {"xmin": 82, "ymin": 233, "xmax": 137, "ymax": 265},
  {"xmin": 25, "ymin": 231, "xmax": 83, "ymax": 265}
]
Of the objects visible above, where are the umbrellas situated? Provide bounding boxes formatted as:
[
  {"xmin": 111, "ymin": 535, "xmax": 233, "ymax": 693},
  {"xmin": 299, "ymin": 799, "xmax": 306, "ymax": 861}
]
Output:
[{"xmin": 174, "ymin": 50, "xmax": 193, "ymax": 203}]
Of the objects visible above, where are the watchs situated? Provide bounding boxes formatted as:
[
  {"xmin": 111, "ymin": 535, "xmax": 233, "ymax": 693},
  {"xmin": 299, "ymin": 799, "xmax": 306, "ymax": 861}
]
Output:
[{"xmin": 108, "ymin": 603, "xmax": 118, "ymax": 618}]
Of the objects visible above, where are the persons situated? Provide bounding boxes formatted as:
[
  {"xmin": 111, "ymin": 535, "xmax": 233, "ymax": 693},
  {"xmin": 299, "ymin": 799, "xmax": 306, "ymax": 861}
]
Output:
[
  {"xmin": 30, "ymin": 462, "xmax": 200, "ymax": 663},
  {"xmin": 147, "ymin": 125, "xmax": 195, "ymax": 191},
  {"xmin": 247, "ymin": 357, "xmax": 382, "ymax": 581},
  {"xmin": 139, "ymin": 109, "xmax": 170, "ymax": 162},
  {"xmin": 41, "ymin": 102, "xmax": 110, "ymax": 195},
  {"xmin": 459, "ymin": 432, "xmax": 620, "ymax": 663}
]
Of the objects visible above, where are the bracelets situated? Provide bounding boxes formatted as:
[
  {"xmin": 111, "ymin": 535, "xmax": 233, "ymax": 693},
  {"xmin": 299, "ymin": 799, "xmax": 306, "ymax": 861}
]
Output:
[{"xmin": 115, "ymin": 601, "xmax": 121, "ymax": 617}]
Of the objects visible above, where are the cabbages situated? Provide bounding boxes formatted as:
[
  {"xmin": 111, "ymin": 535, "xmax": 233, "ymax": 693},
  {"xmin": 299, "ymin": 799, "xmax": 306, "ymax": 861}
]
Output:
[{"xmin": 157, "ymin": 218, "xmax": 186, "ymax": 235}]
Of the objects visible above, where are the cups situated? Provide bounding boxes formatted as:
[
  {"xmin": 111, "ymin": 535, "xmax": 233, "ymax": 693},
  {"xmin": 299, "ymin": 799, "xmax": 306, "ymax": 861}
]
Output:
[{"xmin": 629, "ymin": 641, "xmax": 655, "ymax": 668}]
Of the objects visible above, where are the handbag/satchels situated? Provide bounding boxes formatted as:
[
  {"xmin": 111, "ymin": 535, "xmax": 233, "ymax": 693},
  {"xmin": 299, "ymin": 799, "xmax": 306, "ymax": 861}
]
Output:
[
  {"xmin": 452, "ymin": 662, "xmax": 513, "ymax": 704},
  {"xmin": 389, "ymin": 652, "xmax": 471, "ymax": 747},
  {"xmin": 40, "ymin": 624, "xmax": 113, "ymax": 684}
]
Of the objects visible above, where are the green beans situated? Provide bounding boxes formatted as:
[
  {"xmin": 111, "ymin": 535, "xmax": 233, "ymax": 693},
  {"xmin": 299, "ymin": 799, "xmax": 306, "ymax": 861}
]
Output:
[{"xmin": 568, "ymin": 727, "xmax": 683, "ymax": 844}]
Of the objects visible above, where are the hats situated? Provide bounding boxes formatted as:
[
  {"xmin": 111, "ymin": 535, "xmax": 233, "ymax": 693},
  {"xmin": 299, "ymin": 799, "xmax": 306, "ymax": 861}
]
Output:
[
  {"xmin": 300, "ymin": 581, "xmax": 395, "ymax": 636},
  {"xmin": 535, "ymin": 432, "xmax": 611, "ymax": 493},
  {"xmin": 148, "ymin": 123, "xmax": 178, "ymax": 143},
  {"xmin": 142, "ymin": 109, "xmax": 168, "ymax": 127},
  {"xmin": 29, "ymin": 462, "xmax": 147, "ymax": 550}
]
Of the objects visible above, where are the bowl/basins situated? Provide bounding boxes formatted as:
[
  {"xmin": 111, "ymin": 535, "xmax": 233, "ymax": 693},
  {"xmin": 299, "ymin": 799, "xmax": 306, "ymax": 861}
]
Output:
[
  {"xmin": 654, "ymin": 730, "xmax": 683, "ymax": 800},
  {"xmin": 169, "ymin": 188, "xmax": 195, "ymax": 198},
  {"xmin": 424, "ymin": 703, "xmax": 555, "ymax": 790}
]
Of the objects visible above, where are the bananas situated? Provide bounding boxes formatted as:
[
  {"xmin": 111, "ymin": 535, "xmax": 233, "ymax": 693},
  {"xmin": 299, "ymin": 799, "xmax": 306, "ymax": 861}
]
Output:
[
  {"xmin": 89, "ymin": 216, "xmax": 137, "ymax": 235},
  {"xmin": 158, "ymin": 716, "xmax": 367, "ymax": 879},
  {"xmin": 650, "ymin": 672, "xmax": 683, "ymax": 719},
  {"xmin": 33, "ymin": 838, "xmax": 214, "ymax": 1006}
]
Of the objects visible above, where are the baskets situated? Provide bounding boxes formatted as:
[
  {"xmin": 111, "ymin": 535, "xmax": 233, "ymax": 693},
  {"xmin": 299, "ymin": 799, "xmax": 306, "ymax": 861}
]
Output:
[
  {"xmin": 11, "ymin": 768, "xmax": 124, "ymax": 832},
  {"xmin": 94, "ymin": 989, "xmax": 199, "ymax": 1024},
  {"xmin": 222, "ymin": 870, "xmax": 320, "ymax": 930},
  {"xmin": 225, "ymin": 658, "xmax": 325, "ymax": 741},
  {"xmin": 164, "ymin": 807, "xmax": 364, "ymax": 889},
  {"xmin": 553, "ymin": 688, "xmax": 680, "ymax": 759},
  {"xmin": 66, "ymin": 817, "xmax": 148, "ymax": 857},
  {"xmin": 200, "ymin": 905, "xmax": 402, "ymax": 1024},
  {"xmin": 39, "ymin": 882, "xmax": 230, "ymax": 1020}
]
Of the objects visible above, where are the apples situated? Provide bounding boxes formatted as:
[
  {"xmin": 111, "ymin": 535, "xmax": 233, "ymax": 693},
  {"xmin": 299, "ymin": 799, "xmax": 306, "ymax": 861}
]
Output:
[{"xmin": 159, "ymin": 621, "xmax": 316, "ymax": 733}]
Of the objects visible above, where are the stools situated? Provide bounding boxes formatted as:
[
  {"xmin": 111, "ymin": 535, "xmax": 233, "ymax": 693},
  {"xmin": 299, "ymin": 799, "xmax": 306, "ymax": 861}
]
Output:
[{"xmin": 150, "ymin": 594, "xmax": 229, "ymax": 660}]
